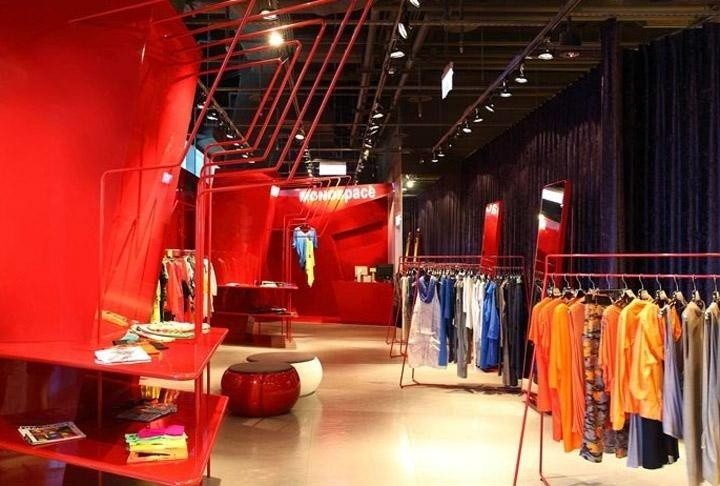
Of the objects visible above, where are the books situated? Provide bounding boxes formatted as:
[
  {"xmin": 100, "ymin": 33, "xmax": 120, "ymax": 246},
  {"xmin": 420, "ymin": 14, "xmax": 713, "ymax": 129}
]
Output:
[{"xmin": 17, "ymin": 418, "xmax": 87, "ymax": 446}]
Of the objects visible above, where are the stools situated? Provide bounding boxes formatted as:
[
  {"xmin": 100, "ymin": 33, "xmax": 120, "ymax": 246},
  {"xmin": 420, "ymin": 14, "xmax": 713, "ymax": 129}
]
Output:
[{"xmin": 222, "ymin": 351, "xmax": 324, "ymax": 419}]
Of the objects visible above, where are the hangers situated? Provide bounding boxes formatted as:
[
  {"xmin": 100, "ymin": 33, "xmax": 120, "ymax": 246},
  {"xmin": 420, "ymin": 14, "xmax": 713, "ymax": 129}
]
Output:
[
  {"xmin": 546, "ymin": 274, "xmax": 720, "ymax": 304},
  {"xmin": 420, "ymin": 264, "xmax": 523, "ymax": 282}
]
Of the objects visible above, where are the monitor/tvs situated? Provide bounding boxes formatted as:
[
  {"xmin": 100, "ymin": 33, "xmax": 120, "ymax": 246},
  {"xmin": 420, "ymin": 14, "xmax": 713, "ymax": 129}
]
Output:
[{"xmin": 376, "ymin": 264, "xmax": 393, "ymax": 279}]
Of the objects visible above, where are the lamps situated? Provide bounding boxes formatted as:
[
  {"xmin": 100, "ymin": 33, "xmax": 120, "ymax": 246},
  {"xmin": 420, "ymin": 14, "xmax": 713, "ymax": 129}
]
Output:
[
  {"xmin": 371, "ymin": 18, "xmax": 413, "ymax": 120},
  {"xmin": 460, "ymin": 38, "xmax": 554, "ymax": 134}
]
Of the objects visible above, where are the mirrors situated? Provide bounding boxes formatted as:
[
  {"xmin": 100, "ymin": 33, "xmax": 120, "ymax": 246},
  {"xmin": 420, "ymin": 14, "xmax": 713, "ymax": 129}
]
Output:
[{"xmin": 532, "ymin": 178, "xmax": 572, "ymax": 299}]
